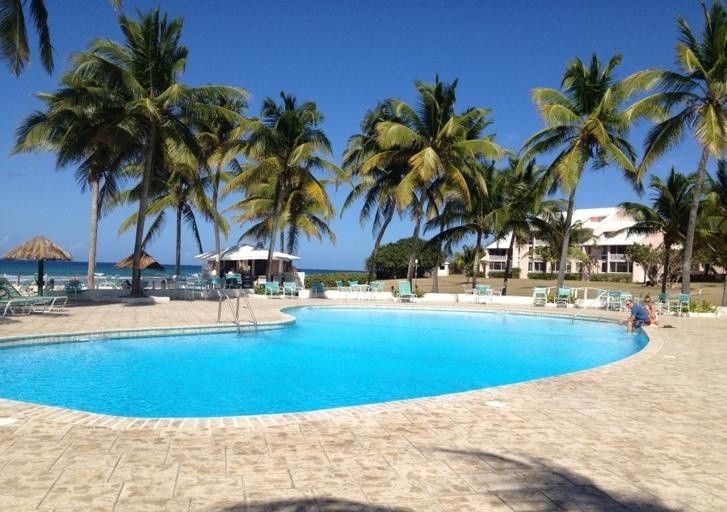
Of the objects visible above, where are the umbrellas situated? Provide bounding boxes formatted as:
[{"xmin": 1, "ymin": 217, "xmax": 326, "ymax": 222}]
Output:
[
  {"xmin": 4, "ymin": 236, "xmax": 71, "ymax": 296},
  {"xmin": 111, "ymin": 250, "xmax": 164, "ymax": 279}
]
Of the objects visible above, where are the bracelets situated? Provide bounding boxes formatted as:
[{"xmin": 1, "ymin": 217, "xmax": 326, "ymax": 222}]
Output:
[{"xmin": 622, "ymin": 320, "xmax": 625, "ymax": 323}]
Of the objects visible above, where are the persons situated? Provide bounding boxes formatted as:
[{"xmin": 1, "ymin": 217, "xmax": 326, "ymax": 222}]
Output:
[
  {"xmin": 642, "ymin": 296, "xmax": 655, "ymax": 324},
  {"xmin": 219, "ymin": 269, "xmax": 226, "ymax": 287},
  {"xmin": 120, "ymin": 280, "xmax": 132, "ymax": 289},
  {"xmin": 617, "ymin": 300, "xmax": 650, "ymax": 333},
  {"xmin": 224, "ymin": 265, "xmax": 237, "ymax": 275},
  {"xmin": 210, "ymin": 267, "xmax": 216, "ymax": 275}
]
